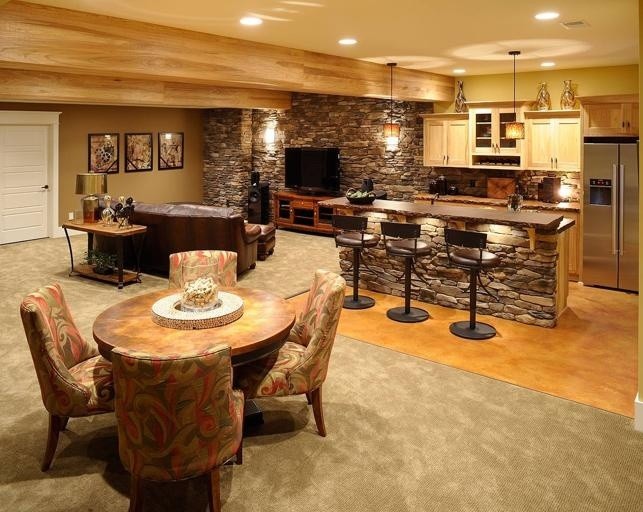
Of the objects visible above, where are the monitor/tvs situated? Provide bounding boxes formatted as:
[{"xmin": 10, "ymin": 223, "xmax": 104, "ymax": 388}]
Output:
[{"xmin": 285, "ymin": 147, "xmax": 340, "ymax": 196}]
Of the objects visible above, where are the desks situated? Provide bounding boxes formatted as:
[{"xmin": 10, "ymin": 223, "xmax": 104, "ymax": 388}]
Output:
[{"xmin": 62, "ymin": 221, "xmax": 148, "ymax": 289}]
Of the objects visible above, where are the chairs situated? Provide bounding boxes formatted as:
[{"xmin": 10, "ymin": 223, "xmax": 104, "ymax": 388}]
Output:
[{"xmin": 331, "ymin": 215, "xmax": 501, "ymax": 339}]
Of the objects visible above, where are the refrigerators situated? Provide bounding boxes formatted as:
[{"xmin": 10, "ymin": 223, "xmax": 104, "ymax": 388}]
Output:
[{"xmin": 581, "ymin": 141, "xmax": 639, "ymax": 293}]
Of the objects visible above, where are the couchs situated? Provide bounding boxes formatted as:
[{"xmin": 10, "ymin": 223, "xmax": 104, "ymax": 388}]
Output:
[{"xmin": 82, "ymin": 195, "xmax": 275, "ymax": 278}]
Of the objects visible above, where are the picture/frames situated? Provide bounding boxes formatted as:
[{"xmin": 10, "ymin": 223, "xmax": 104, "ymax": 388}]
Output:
[{"xmin": 87, "ymin": 133, "xmax": 184, "ymax": 175}]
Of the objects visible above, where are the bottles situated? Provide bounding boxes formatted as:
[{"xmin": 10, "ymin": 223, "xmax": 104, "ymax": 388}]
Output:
[
  {"xmin": 429, "ymin": 173, "xmax": 459, "ymax": 196},
  {"xmin": 560, "ymin": 80, "xmax": 577, "ymax": 111},
  {"xmin": 455, "ymin": 80, "xmax": 468, "ymax": 113},
  {"xmin": 101, "ymin": 196, "xmax": 115, "ymax": 226},
  {"xmin": 114, "ymin": 197, "xmax": 130, "ymax": 227},
  {"xmin": 535, "ymin": 82, "xmax": 553, "ymax": 112}
]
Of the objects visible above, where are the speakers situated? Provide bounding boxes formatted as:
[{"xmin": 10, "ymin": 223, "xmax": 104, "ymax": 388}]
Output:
[
  {"xmin": 247, "ymin": 183, "xmax": 270, "ymax": 225},
  {"xmin": 251, "ymin": 171, "xmax": 259, "ymax": 185}
]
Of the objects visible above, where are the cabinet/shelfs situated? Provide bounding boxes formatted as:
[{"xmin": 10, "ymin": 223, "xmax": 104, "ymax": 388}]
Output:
[
  {"xmin": 418, "ymin": 93, "xmax": 639, "ymax": 173},
  {"xmin": 271, "ymin": 192, "xmax": 341, "ymax": 237}
]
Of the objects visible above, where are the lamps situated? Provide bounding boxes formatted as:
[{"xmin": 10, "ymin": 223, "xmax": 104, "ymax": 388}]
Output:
[
  {"xmin": 75, "ymin": 174, "xmax": 108, "ymax": 223},
  {"xmin": 506, "ymin": 51, "xmax": 525, "ymax": 140},
  {"xmin": 383, "ymin": 63, "xmax": 400, "ymax": 139}
]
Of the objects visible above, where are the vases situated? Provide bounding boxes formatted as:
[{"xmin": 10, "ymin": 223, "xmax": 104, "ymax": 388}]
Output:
[
  {"xmin": 535, "ymin": 79, "xmax": 577, "ymax": 111},
  {"xmin": 455, "ymin": 81, "xmax": 466, "ymax": 113}
]
